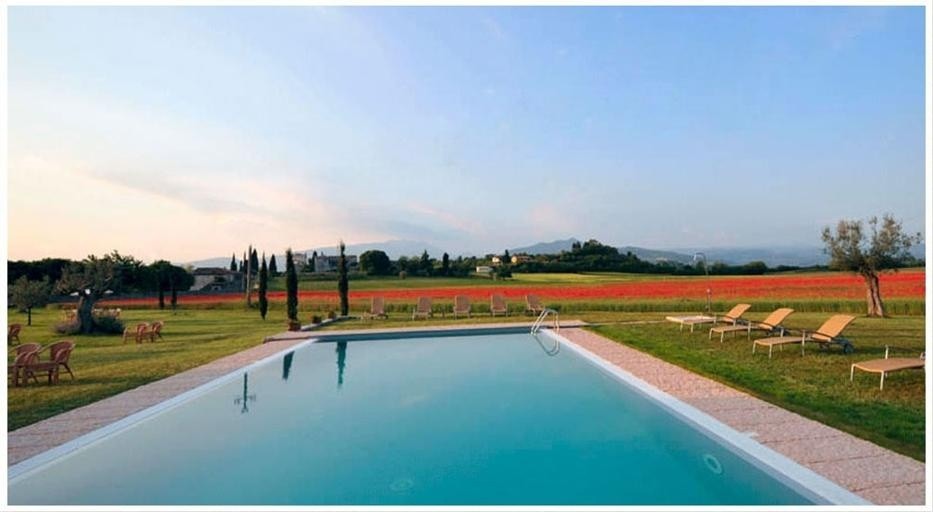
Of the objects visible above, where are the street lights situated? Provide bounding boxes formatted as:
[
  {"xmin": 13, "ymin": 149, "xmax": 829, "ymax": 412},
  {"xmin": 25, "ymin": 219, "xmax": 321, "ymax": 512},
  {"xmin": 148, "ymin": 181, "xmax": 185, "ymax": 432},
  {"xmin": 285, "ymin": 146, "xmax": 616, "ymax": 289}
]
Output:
[{"xmin": 694, "ymin": 253, "xmax": 711, "ymax": 311}]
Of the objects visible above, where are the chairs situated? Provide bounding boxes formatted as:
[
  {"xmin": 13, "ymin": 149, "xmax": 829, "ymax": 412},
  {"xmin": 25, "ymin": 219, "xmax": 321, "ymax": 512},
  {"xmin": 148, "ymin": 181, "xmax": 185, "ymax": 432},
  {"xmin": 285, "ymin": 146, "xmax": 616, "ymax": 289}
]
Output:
[
  {"xmin": 8, "ymin": 324, "xmax": 76, "ymax": 387},
  {"xmin": 680, "ymin": 302, "xmax": 925, "ymax": 391},
  {"xmin": 123, "ymin": 320, "xmax": 164, "ymax": 344},
  {"xmin": 365, "ymin": 292, "xmax": 546, "ymax": 320}
]
